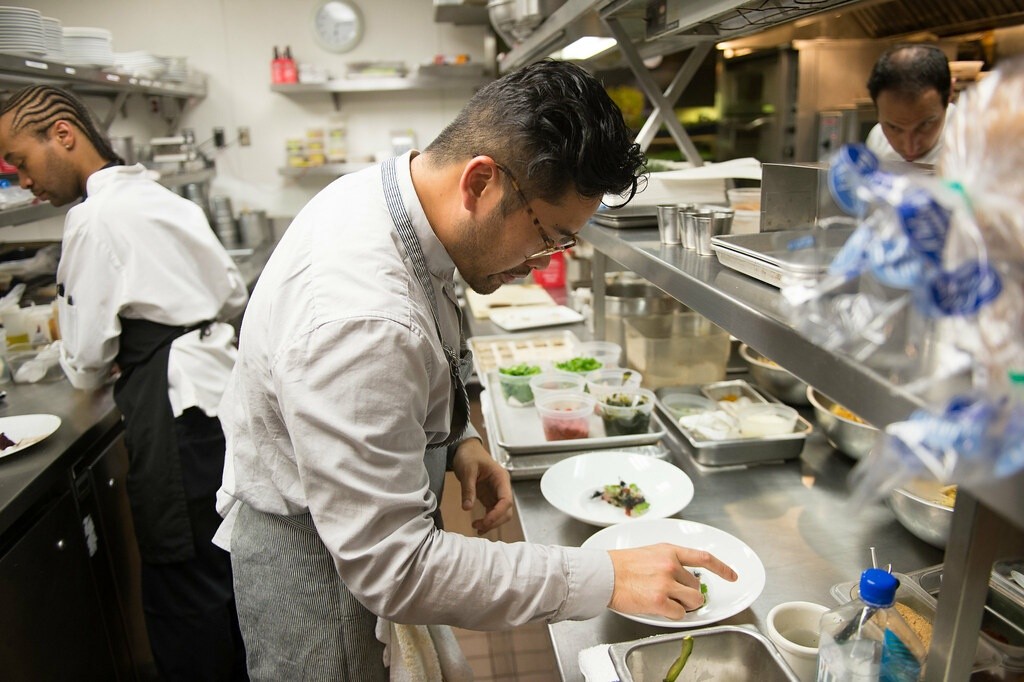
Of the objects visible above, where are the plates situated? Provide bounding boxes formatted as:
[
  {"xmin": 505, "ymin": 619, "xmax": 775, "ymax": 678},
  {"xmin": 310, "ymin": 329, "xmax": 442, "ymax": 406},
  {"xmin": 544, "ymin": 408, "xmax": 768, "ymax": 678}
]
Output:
[
  {"xmin": 0, "ymin": 414, "xmax": 62, "ymax": 458},
  {"xmin": 581, "ymin": 518, "xmax": 766, "ymax": 627},
  {"xmin": 0, "ymin": 6, "xmax": 188, "ymax": 83},
  {"xmin": 465, "ymin": 284, "xmax": 584, "ymax": 330},
  {"xmin": 540, "ymin": 451, "xmax": 695, "ymax": 528}
]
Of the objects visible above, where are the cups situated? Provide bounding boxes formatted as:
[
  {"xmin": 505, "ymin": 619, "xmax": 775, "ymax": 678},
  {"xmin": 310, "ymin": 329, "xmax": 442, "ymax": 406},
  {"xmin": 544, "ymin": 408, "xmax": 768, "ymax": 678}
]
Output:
[
  {"xmin": 678, "ymin": 210, "xmax": 717, "ymax": 249},
  {"xmin": 692, "ymin": 213, "xmax": 734, "ymax": 256},
  {"xmin": 656, "ymin": 203, "xmax": 693, "ymax": 245},
  {"xmin": 767, "ymin": 601, "xmax": 832, "ymax": 682},
  {"xmin": 0, "ymin": 328, "xmax": 11, "ymax": 384}
]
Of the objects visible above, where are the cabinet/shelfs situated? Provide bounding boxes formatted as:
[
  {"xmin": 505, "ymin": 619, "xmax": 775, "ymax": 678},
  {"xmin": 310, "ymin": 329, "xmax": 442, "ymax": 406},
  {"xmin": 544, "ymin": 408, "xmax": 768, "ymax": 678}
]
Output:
[
  {"xmin": 0, "ymin": 52, "xmax": 219, "ymax": 229},
  {"xmin": 270, "ymin": 74, "xmax": 493, "ymax": 177}
]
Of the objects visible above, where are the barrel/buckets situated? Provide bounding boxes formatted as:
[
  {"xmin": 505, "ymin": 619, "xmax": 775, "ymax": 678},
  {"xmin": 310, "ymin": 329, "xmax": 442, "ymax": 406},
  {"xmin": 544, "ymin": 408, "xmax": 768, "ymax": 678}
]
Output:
[
  {"xmin": 240, "ymin": 213, "xmax": 270, "ymax": 245},
  {"xmin": 591, "ymin": 285, "xmax": 673, "ymax": 369}
]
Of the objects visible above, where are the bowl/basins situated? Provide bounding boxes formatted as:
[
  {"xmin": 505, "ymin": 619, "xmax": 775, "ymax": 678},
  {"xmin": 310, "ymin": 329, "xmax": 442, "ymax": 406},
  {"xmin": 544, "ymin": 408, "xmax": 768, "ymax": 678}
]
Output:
[
  {"xmin": 727, "ymin": 188, "xmax": 761, "ymax": 210},
  {"xmin": 948, "ymin": 61, "xmax": 984, "ymax": 81},
  {"xmin": 549, "ymin": 341, "xmax": 655, "ymax": 437},
  {"xmin": 866, "ymin": 442, "xmax": 959, "ymax": 548},
  {"xmin": 664, "ymin": 393, "xmax": 715, "ymax": 424},
  {"xmin": 739, "ymin": 344, "xmax": 808, "ymax": 405},
  {"xmin": 737, "ymin": 405, "xmax": 798, "ymax": 438},
  {"xmin": 530, "ymin": 372, "xmax": 595, "ymax": 441},
  {"xmin": 496, "ymin": 359, "xmax": 541, "ymax": 406},
  {"xmin": 807, "ymin": 386, "xmax": 880, "ymax": 460}
]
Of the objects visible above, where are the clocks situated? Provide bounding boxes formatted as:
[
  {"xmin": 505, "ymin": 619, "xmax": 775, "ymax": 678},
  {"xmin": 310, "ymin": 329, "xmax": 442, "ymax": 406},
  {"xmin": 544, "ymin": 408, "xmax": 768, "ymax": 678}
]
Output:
[{"xmin": 308, "ymin": 0, "xmax": 365, "ymax": 53}]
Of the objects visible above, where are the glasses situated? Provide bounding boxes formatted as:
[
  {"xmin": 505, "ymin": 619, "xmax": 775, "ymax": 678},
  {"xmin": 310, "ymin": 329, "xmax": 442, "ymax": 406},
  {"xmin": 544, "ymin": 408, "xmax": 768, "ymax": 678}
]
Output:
[{"xmin": 488, "ymin": 160, "xmax": 578, "ymax": 263}]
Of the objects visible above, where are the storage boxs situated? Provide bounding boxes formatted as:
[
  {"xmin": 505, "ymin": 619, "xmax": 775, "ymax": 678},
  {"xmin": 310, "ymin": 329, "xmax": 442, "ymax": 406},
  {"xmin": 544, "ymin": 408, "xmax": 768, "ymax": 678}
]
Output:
[{"xmin": 622, "ymin": 311, "xmax": 732, "ymax": 387}]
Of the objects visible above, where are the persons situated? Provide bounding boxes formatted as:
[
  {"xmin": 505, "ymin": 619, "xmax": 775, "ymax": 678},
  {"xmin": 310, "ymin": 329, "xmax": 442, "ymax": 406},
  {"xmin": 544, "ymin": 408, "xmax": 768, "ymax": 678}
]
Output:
[
  {"xmin": 230, "ymin": 61, "xmax": 739, "ymax": 682},
  {"xmin": 0, "ymin": 83, "xmax": 248, "ymax": 682},
  {"xmin": 867, "ymin": 44, "xmax": 957, "ymax": 174}
]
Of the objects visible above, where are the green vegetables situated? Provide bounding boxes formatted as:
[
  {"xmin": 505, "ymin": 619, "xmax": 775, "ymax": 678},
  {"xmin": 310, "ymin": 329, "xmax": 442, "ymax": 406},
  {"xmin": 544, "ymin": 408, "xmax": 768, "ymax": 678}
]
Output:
[
  {"xmin": 701, "ymin": 582, "xmax": 707, "ymax": 604},
  {"xmin": 498, "ymin": 355, "xmax": 603, "ymax": 405},
  {"xmin": 606, "ymin": 482, "xmax": 649, "ymax": 511}
]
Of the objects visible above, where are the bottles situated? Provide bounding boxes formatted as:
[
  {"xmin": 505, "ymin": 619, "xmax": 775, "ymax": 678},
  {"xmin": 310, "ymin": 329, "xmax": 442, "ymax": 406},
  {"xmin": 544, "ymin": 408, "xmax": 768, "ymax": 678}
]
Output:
[
  {"xmin": 272, "ymin": 47, "xmax": 296, "ymax": 84},
  {"xmin": 818, "ymin": 569, "xmax": 926, "ymax": 682},
  {"xmin": 32, "ymin": 304, "xmax": 59, "ymax": 353}
]
